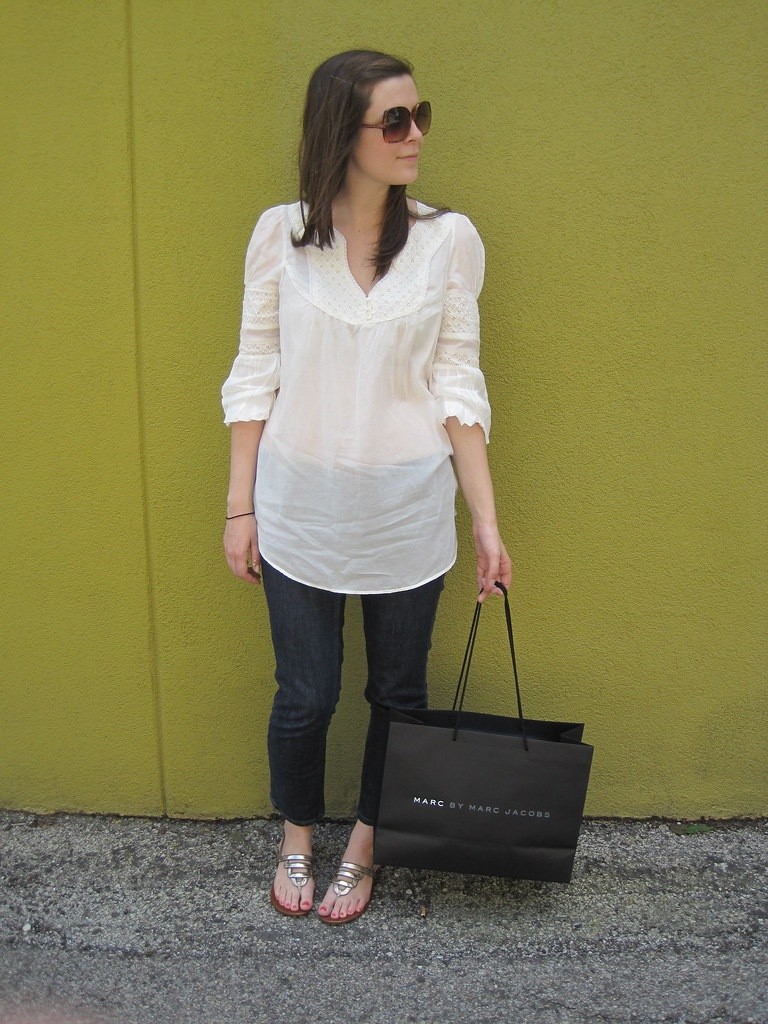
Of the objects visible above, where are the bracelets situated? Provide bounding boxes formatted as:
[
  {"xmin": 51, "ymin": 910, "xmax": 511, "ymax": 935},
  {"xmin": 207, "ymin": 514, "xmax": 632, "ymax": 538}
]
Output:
[{"xmin": 225, "ymin": 512, "xmax": 255, "ymax": 520}]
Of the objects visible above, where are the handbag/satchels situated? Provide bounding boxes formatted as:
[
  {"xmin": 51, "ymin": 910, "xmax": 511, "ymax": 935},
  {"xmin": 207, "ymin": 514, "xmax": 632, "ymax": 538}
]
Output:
[{"xmin": 373, "ymin": 581, "xmax": 594, "ymax": 883}]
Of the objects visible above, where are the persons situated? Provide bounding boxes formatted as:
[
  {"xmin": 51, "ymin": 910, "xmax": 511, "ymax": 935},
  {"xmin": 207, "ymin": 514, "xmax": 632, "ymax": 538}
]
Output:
[{"xmin": 219, "ymin": 48, "xmax": 513, "ymax": 926}]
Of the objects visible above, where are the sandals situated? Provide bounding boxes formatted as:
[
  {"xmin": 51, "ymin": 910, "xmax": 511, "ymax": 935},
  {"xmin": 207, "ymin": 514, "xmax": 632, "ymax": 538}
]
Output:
[
  {"xmin": 318, "ymin": 862, "xmax": 381, "ymax": 925},
  {"xmin": 271, "ymin": 827, "xmax": 315, "ymax": 915}
]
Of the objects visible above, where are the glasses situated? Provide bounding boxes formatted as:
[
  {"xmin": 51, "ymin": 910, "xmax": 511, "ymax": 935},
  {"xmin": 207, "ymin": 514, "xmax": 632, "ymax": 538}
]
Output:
[{"xmin": 359, "ymin": 101, "xmax": 432, "ymax": 143}]
